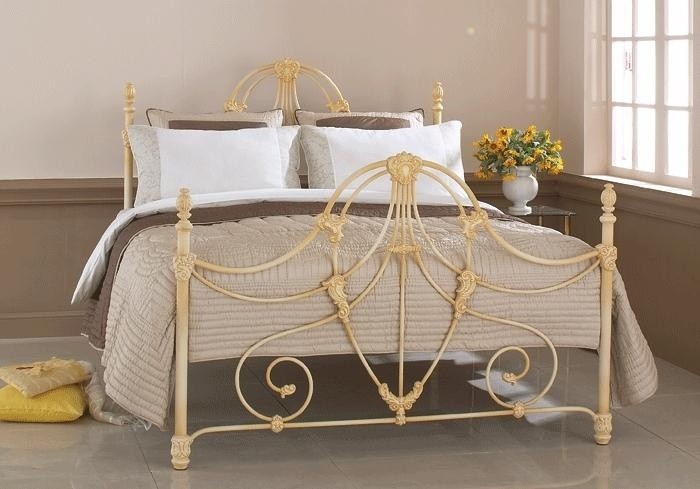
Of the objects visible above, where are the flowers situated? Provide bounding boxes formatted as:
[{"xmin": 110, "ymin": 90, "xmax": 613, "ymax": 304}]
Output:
[{"xmin": 472, "ymin": 119, "xmax": 565, "ymax": 183}]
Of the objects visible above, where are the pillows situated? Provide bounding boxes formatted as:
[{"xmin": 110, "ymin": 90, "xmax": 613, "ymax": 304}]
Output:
[
  {"xmin": 297, "ymin": 117, "xmax": 469, "ymax": 205},
  {"xmin": 127, "ymin": 123, "xmax": 304, "ymax": 207},
  {"xmin": 143, "ymin": 105, "xmax": 285, "ymax": 133},
  {"xmin": 294, "ymin": 107, "xmax": 428, "ymax": 131},
  {"xmin": 0, "ymin": 384, "xmax": 88, "ymax": 424}
]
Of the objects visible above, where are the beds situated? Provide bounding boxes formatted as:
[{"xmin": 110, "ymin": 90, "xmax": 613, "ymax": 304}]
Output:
[{"xmin": 120, "ymin": 56, "xmax": 618, "ymax": 471}]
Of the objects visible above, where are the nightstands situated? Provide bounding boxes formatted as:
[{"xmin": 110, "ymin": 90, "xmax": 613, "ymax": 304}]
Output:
[{"xmin": 488, "ymin": 200, "xmax": 577, "ymax": 236}]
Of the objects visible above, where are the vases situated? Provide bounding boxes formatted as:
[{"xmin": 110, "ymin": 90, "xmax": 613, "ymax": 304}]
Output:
[{"xmin": 501, "ymin": 165, "xmax": 539, "ymax": 216}]
